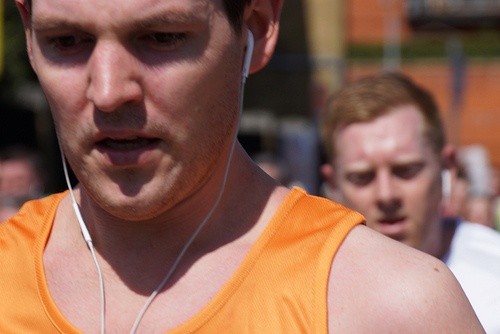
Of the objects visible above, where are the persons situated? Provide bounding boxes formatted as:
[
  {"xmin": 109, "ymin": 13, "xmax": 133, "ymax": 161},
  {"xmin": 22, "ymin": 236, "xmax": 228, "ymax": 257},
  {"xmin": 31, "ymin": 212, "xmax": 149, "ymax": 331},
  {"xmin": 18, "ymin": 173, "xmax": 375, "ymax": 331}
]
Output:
[
  {"xmin": 254, "ymin": 153, "xmax": 286, "ymax": 192},
  {"xmin": 0, "ymin": 154, "xmax": 41, "ymax": 226},
  {"xmin": 321, "ymin": 70, "xmax": 500, "ymax": 334},
  {"xmin": 0, "ymin": 0, "xmax": 485, "ymax": 334}
]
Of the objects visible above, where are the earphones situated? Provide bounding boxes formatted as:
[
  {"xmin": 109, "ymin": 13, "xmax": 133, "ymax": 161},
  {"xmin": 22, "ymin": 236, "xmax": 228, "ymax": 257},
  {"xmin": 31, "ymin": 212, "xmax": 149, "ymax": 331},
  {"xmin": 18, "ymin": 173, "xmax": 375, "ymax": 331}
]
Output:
[{"xmin": 240, "ymin": 30, "xmax": 254, "ymax": 84}]
